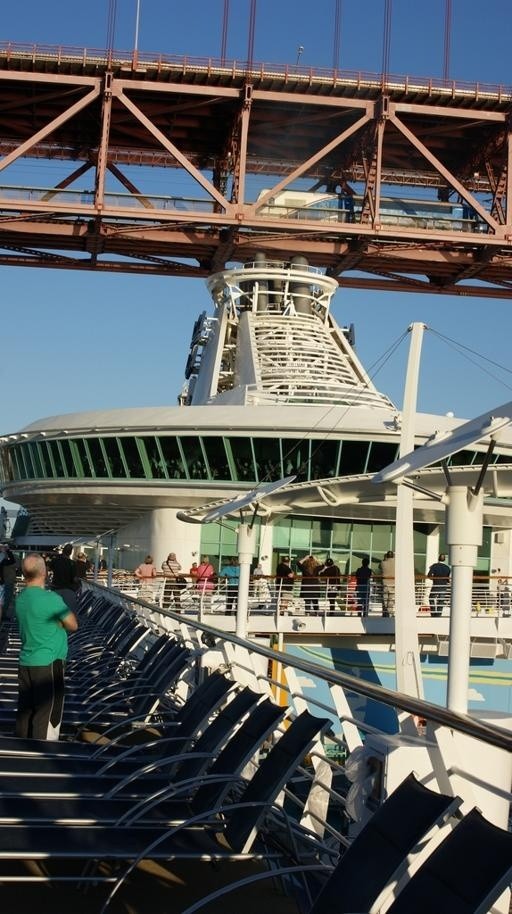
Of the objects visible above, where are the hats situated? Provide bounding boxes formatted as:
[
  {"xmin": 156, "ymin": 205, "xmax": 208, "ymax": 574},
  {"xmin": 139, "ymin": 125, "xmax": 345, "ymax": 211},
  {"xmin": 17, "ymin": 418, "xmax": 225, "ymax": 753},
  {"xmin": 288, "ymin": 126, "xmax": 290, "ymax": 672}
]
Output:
[{"xmin": 325, "ymin": 558, "xmax": 334, "ymax": 566}]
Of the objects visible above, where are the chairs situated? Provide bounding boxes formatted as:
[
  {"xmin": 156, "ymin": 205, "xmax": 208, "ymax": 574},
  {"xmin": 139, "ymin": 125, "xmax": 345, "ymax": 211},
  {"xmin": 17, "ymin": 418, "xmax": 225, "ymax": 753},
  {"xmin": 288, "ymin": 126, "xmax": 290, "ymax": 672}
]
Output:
[
  {"xmin": 180, "ymin": 771, "xmax": 464, "ymax": 912},
  {"xmin": 380, "ymin": 807, "xmax": 512, "ymax": 914},
  {"xmin": 2, "ymin": 709, "xmax": 333, "ymax": 911},
  {"xmin": 2, "ymin": 586, "xmax": 290, "ymax": 893}
]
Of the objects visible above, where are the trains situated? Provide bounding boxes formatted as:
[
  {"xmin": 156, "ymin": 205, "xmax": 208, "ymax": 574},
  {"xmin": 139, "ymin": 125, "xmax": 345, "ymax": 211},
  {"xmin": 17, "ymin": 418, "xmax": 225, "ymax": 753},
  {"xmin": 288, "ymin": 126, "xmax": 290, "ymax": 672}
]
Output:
[{"xmin": 254, "ymin": 188, "xmax": 505, "ymax": 232}]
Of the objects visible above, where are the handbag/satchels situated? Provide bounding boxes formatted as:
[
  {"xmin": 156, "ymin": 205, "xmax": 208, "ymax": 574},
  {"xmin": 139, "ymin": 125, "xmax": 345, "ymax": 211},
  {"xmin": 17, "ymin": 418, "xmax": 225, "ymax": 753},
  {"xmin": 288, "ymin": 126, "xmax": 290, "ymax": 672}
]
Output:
[{"xmin": 176, "ymin": 577, "xmax": 186, "ymax": 589}]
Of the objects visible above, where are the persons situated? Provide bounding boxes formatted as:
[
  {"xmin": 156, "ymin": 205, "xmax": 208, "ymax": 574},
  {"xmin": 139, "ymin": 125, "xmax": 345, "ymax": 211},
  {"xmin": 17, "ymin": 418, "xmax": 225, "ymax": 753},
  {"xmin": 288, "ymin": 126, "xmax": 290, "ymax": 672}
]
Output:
[
  {"xmin": 338, "ymin": 178, "xmax": 359, "ymax": 224},
  {"xmin": 427, "ymin": 554, "xmax": 451, "ymax": 617},
  {"xmin": 49, "ymin": 544, "xmax": 111, "ymax": 623},
  {"xmin": 15, "ymin": 553, "xmax": 82, "ymax": 746},
  {"xmin": 166, "ymin": 458, "xmax": 319, "ymax": 483},
  {"xmin": 137, "ymin": 551, "xmax": 395, "ymax": 618},
  {"xmin": 0, "ymin": 547, "xmax": 17, "ymax": 651}
]
